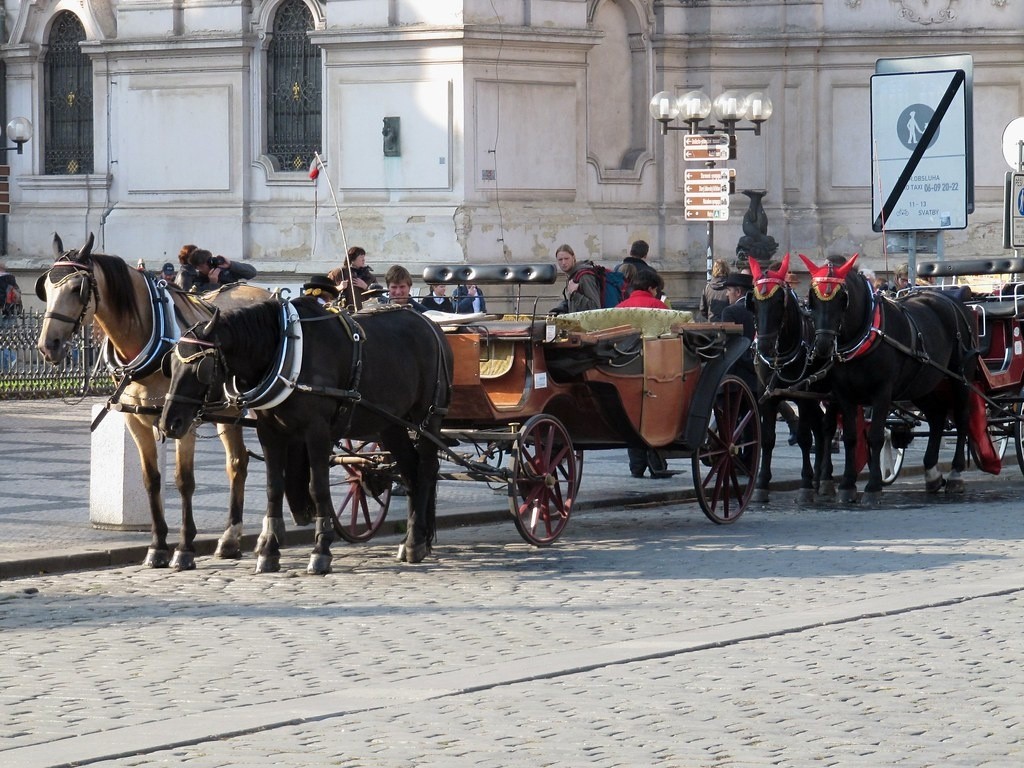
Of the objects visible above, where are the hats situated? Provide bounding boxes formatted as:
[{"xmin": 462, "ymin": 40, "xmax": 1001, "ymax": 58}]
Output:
[
  {"xmin": 303, "ymin": 275, "xmax": 339, "ymax": 298},
  {"xmin": 163, "ymin": 263, "xmax": 174, "ymax": 275},
  {"xmin": 785, "ymin": 273, "xmax": 801, "ymax": 283}
]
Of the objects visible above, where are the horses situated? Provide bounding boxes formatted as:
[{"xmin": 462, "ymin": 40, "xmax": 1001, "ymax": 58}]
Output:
[
  {"xmin": 161, "ymin": 293, "xmax": 456, "ymax": 574},
  {"xmin": 746, "ymin": 251, "xmax": 984, "ymax": 506},
  {"xmin": 35, "ymin": 232, "xmax": 279, "ymax": 572}
]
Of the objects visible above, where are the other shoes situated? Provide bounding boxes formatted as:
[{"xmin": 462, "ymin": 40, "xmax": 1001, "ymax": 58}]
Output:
[
  {"xmin": 631, "ymin": 467, "xmax": 644, "ymax": 478},
  {"xmin": 391, "ymin": 482, "xmax": 407, "ymax": 495},
  {"xmin": 810, "ymin": 439, "xmax": 840, "ymax": 454},
  {"xmin": 788, "ymin": 433, "xmax": 798, "ymax": 447},
  {"xmin": 651, "ymin": 471, "xmax": 675, "ymax": 480}
]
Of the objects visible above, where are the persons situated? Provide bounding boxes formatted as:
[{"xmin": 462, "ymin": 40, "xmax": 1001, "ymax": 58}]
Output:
[
  {"xmin": 549, "ymin": 245, "xmax": 602, "ymax": 316},
  {"xmin": 303, "ymin": 275, "xmax": 338, "ymax": 303},
  {"xmin": 701, "ymin": 260, "xmax": 802, "ymax": 475},
  {"xmin": 614, "ymin": 240, "xmax": 671, "ymax": 309},
  {"xmin": 329, "ymin": 248, "xmax": 377, "ymax": 315},
  {"xmin": 421, "ymin": 285, "xmax": 488, "ymax": 314},
  {"xmin": 0, "ymin": 266, "xmax": 21, "ymax": 372},
  {"xmin": 158, "ymin": 247, "xmax": 258, "ymax": 293},
  {"xmin": 386, "ymin": 265, "xmax": 427, "ymax": 496},
  {"xmin": 810, "ymin": 255, "xmax": 936, "ymax": 452}
]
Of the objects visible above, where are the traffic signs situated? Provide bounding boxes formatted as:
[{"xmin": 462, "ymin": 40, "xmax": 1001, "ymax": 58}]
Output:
[
  {"xmin": 684, "ymin": 195, "xmax": 730, "ymax": 208},
  {"xmin": 684, "ymin": 207, "xmax": 730, "ymax": 221},
  {"xmin": 684, "ymin": 168, "xmax": 730, "ymax": 182},
  {"xmin": 683, "ymin": 146, "xmax": 729, "ymax": 160},
  {"xmin": 683, "ymin": 181, "xmax": 730, "ymax": 193},
  {"xmin": 684, "ymin": 133, "xmax": 729, "ymax": 146}
]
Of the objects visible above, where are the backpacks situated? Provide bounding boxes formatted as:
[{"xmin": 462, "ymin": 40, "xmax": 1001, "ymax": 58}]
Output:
[{"xmin": 572, "ymin": 261, "xmax": 629, "ymax": 309}]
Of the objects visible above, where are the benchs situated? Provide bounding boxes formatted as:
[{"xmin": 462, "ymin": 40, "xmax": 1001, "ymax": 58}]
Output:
[
  {"xmin": 558, "ymin": 308, "xmax": 699, "ymax": 375},
  {"xmin": 456, "ymin": 319, "xmax": 546, "ymax": 342},
  {"xmin": 895, "ymin": 257, "xmax": 1024, "ymax": 324}
]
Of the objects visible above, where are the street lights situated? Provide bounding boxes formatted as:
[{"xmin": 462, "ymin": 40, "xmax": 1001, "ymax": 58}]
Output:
[
  {"xmin": 646, "ymin": 89, "xmax": 775, "ymax": 288},
  {"xmin": 0, "ymin": 118, "xmax": 34, "ymax": 257}
]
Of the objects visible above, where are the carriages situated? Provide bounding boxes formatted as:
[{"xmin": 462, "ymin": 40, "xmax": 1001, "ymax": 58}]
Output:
[
  {"xmin": 730, "ymin": 249, "xmax": 1024, "ymax": 502},
  {"xmin": 35, "ymin": 232, "xmax": 768, "ymax": 582}
]
